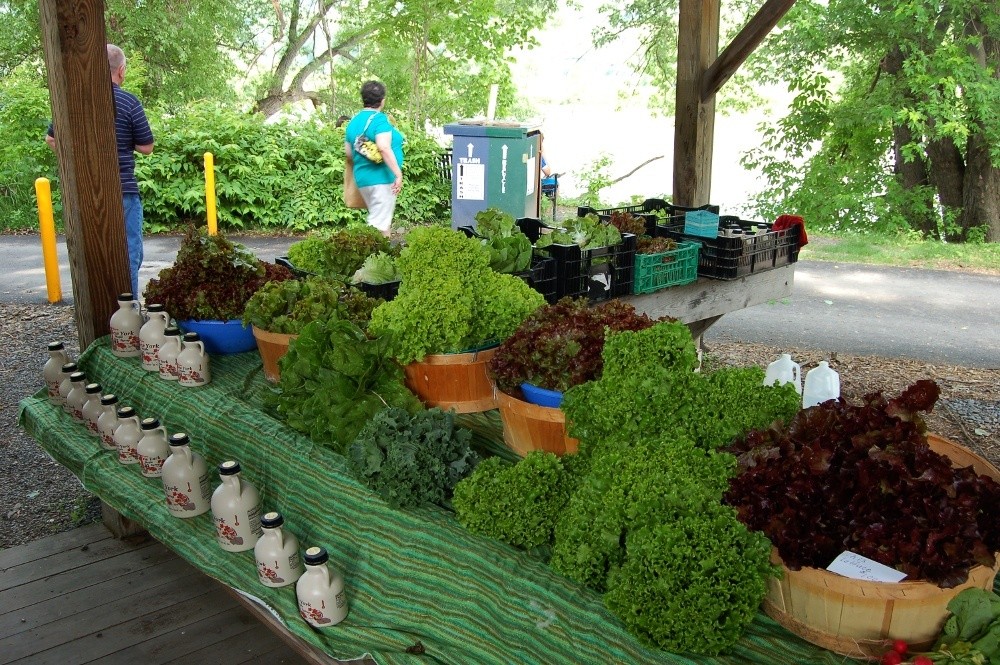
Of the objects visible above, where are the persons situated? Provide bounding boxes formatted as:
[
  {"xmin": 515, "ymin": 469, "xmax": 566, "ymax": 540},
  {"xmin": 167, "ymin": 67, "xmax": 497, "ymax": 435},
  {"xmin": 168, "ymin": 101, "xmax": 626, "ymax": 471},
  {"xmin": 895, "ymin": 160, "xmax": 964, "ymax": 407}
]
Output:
[
  {"xmin": 541, "ymin": 156, "xmax": 552, "ymax": 178},
  {"xmin": 345, "ymin": 79, "xmax": 404, "ymax": 236},
  {"xmin": 334, "ymin": 115, "xmax": 351, "ymax": 130},
  {"xmin": 45, "ymin": 44, "xmax": 154, "ymax": 310}
]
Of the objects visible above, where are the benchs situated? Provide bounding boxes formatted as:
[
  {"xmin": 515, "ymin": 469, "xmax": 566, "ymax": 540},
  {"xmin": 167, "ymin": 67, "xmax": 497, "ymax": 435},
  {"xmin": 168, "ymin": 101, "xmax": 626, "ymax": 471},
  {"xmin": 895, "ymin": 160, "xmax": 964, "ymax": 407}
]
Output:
[{"xmin": 430, "ymin": 148, "xmax": 559, "ymax": 222}]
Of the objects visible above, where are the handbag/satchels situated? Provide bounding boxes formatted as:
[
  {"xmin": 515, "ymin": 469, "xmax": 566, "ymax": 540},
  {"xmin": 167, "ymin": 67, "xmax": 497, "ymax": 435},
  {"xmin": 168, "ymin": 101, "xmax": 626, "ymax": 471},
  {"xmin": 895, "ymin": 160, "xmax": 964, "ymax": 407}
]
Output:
[
  {"xmin": 344, "ymin": 158, "xmax": 367, "ymax": 209},
  {"xmin": 354, "ymin": 113, "xmax": 384, "ymax": 164}
]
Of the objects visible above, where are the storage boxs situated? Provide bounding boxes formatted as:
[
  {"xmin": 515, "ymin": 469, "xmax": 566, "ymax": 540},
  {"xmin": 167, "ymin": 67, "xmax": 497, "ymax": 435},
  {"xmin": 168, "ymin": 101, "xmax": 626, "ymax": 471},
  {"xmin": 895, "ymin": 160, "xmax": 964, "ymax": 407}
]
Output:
[{"xmin": 457, "ymin": 198, "xmax": 801, "ymax": 306}]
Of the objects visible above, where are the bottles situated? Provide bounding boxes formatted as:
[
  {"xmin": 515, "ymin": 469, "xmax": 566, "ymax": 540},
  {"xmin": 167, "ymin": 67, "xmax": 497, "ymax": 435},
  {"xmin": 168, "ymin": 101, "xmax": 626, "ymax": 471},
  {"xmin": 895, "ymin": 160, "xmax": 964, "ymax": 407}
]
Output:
[
  {"xmin": 160, "ymin": 432, "xmax": 212, "ymax": 518},
  {"xmin": 43, "ymin": 341, "xmax": 77, "ymax": 405},
  {"xmin": 803, "ymin": 361, "xmax": 840, "ymax": 410},
  {"xmin": 763, "ymin": 354, "xmax": 802, "ymax": 398},
  {"xmin": 139, "ymin": 304, "xmax": 170, "ymax": 371},
  {"xmin": 177, "ymin": 332, "xmax": 211, "ymax": 387},
  {"xmin": 718, "ymin": 224, "xmax": 756, "ymax": 244},
  {"xmin": 137, "ymin": 417, "xmax": 171, "ymax": 478},
  {"xmin": 97, "ymin": 394, "xmax": 122, "ymax": 450},
  {"xmin": 757, "ymin": 224, "xmax": 773, "ymax": 248},
  {"xmin": 296, "ymin": 547, "xmax": 349, "ymax": 627},
  {"xmin": 81, "ymin": 383, "xmax": 104, "ymax": 436},
  {"xmin": 114, "ymin": 406, "xmax": 143, "ymax": 464},
  {"xmin": 58, "ymin": 362, "xmax": 80, "ymax": 414},
  {"xmin": 210, "ymin": 459, "xmax": 265, "ymax": 552},
  {"xmin": 158, "ymin": 327, "xmax": 185, "ymax": 381},
  {"xmin": 254, "ymin": 511, "xmax": 303, "ymax": 588},
  {"xmin": 110, "ymin": 293, "xmax": 146, "ymax": 358},
  {"xmin": 67, "ymin": 372, "xmax": 89, "ymax": 424}
]
